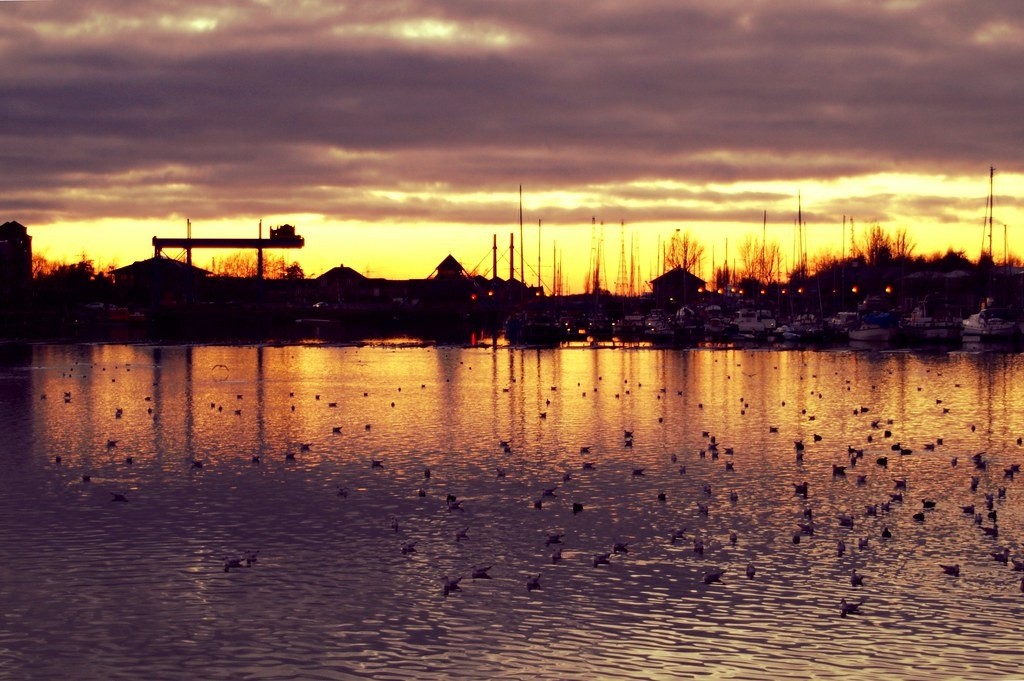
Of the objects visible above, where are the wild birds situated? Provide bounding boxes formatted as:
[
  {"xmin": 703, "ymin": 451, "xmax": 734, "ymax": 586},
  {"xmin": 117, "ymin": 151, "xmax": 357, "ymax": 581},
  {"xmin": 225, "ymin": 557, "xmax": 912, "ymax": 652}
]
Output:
[{"xmin": 41, "ymin": 340, "xmax": 1023, "ymax": 612}]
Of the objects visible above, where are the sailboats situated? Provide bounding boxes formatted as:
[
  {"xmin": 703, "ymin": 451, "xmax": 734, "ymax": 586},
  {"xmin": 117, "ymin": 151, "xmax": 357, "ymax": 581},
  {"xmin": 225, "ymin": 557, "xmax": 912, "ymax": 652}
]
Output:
[{"xmin": 498, "ymin": 164, "xmax": 1022, "ymax": 354}]
